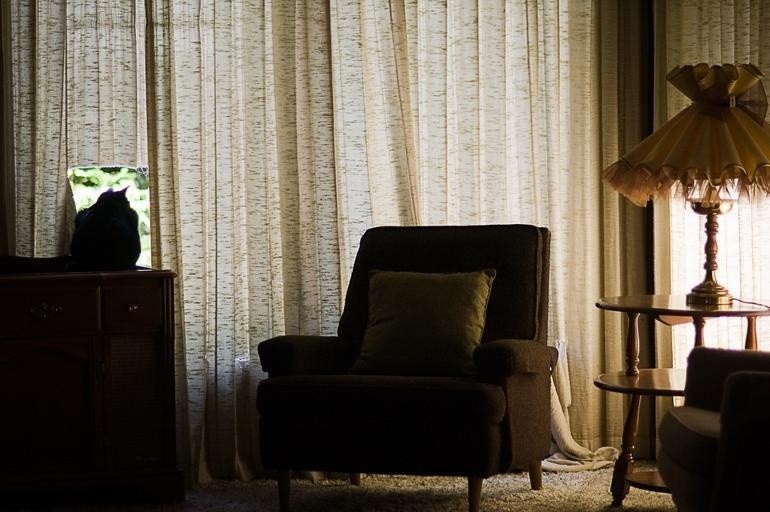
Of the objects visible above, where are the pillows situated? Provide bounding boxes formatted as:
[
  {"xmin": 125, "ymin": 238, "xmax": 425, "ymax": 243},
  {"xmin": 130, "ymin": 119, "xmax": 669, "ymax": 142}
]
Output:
[{"xmin": 350, "ymin": 260, "xmax": 501, "ymax": 380}]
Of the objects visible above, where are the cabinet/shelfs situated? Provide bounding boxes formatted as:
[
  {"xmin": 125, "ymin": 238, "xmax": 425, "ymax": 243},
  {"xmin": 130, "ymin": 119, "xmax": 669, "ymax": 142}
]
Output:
[{"xmin": 1, "ymin": 257, "xmax": 186, "ymax": 511}]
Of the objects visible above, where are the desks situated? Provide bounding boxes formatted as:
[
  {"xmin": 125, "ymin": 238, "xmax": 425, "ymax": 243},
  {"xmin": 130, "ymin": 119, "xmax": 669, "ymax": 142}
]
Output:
[{"xmin": 589, "ymin": 290, "xmax": 770, "ymax": 510}]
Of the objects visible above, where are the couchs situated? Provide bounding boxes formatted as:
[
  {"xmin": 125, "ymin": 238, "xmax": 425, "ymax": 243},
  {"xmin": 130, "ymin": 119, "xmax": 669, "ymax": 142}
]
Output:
[
  {"xmin": 654, "ymin": 337, "xmax": 770, "ymax": 509},
  {"xmin": 255, "ymin": 222, "xmax": 559, "ymax": 510}
]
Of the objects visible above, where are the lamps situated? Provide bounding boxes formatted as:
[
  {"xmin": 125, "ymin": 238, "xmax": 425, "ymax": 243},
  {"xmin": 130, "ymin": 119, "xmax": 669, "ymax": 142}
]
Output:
[{"xmin": 594, "ymin": 54, "xmax": 770, "ymax": 308}]
